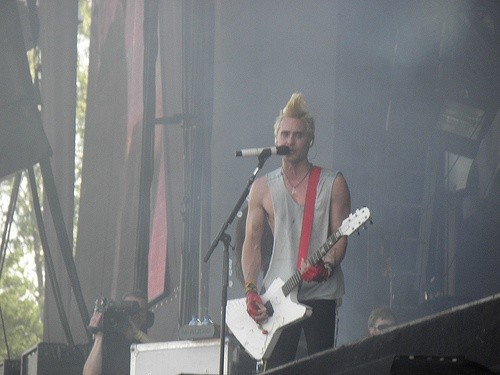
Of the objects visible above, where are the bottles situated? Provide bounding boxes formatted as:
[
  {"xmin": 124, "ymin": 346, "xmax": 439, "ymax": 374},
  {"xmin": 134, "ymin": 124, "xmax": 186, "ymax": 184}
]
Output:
[
  {"xmin": 201, "ymin": 312, "xmax": 216, "ymax": 339},
  {"xmin": 186, "ymin": 313, "xmax": 202, "ymax": 339}
]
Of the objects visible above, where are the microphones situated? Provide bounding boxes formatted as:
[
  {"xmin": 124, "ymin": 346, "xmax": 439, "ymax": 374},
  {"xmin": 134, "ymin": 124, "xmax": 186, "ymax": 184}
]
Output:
[{"xmin": 235, "ymin": 145, "xmax": 289, "ymax": 157}]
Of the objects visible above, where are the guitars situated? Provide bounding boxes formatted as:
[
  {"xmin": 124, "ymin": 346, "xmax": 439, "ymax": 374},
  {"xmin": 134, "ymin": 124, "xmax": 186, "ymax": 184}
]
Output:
[{"xmin": 225, "ymin": 205, "xmax": 373, "ymax": 361}]
